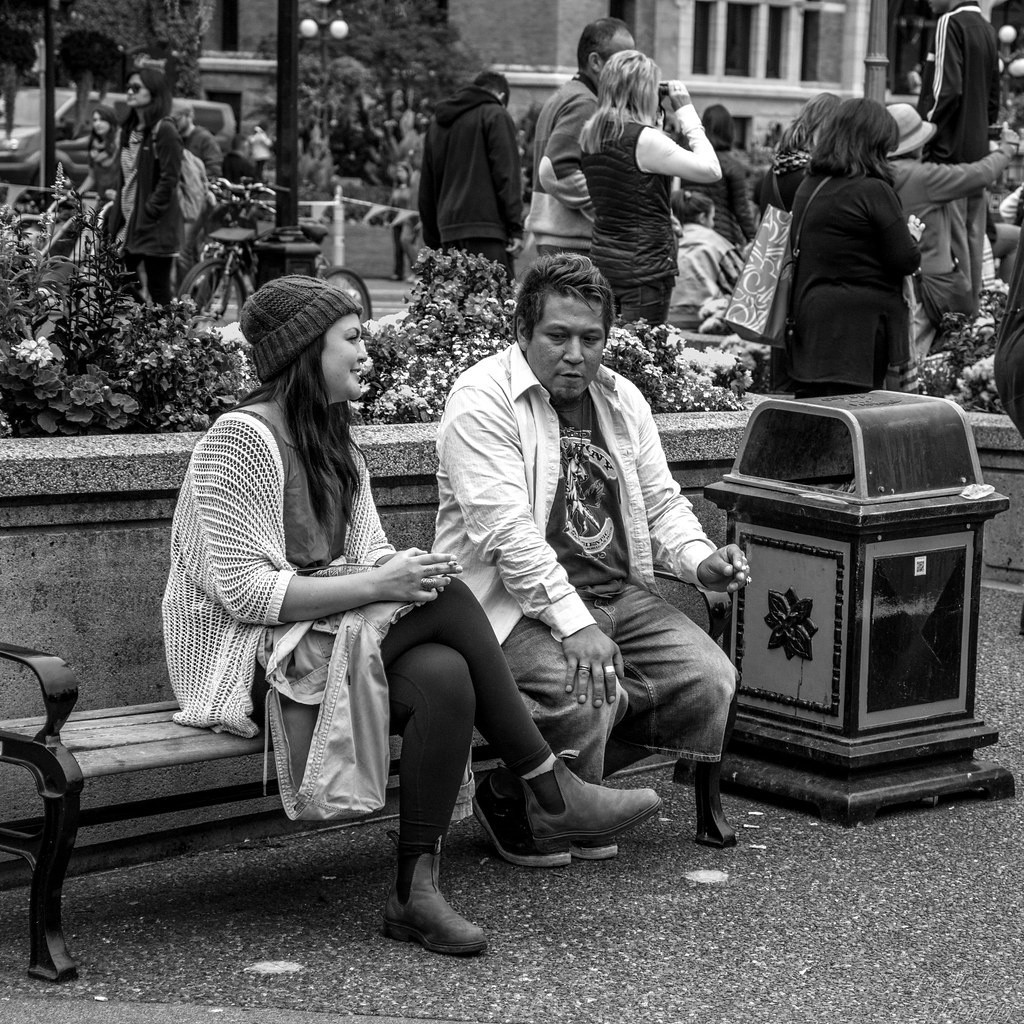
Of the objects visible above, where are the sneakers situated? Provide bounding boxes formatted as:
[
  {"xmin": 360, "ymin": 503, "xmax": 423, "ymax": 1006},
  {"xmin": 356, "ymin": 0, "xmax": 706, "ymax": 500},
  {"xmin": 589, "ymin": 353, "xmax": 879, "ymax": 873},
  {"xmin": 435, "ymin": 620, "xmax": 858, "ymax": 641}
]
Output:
[
  {"xmin": 472, "ymin": 761, "xmax": 570, "ymax": 866},
  {"xmin": 570, "ymin": 841, "xmax": 618, "ymax": 859}
]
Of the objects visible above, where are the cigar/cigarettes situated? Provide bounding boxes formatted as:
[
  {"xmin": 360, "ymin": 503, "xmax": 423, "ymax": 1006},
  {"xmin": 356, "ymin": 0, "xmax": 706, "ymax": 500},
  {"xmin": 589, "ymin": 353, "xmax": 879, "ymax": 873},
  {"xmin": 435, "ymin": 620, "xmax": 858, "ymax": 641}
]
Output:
[
  {"xmin": 447, "ymin": 561, "xmax": 458, "ymax": 566},
  {"xmin": 733, "ymin": 566, "xmax": 747, "ymax": 572}
]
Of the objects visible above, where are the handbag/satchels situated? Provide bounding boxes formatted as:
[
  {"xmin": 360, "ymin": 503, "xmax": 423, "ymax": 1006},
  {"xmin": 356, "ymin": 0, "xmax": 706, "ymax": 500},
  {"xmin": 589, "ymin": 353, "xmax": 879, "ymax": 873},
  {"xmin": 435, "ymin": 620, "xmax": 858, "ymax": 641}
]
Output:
[
  {"xmin": 917, "ymin": 265, "xmax": 973, "ymax": 327},
  {"xmin": 723, "ymin": 205, "xmax": 795, "ymax": 349}
]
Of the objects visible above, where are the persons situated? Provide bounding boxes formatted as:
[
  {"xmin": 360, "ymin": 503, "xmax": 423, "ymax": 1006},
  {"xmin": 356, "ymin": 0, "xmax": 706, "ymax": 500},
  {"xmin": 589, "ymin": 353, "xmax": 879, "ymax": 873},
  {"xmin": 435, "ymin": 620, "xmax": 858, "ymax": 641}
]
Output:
[
  {"xmin": 159, "ymin": 274, "xmax": 664, "ymax": 955},
  {"xmin": 385, "ymin": 161, "xmax": 419, "ymax": 281},
  {"xmin": 418, "ymin": 70, "xmax": 526, "ymax": 284},
  {"xmin": 223, "ymin": 135, "xmax": 263, "ymax": 181},
  {"xmin": 527, "ymin": 16, "xmax": 763, "ymax": 335},
  {"xmin": 107, "ymin": 67, "xmax": 184, "ymax": 314},
  {"xmin": 174, "ymin": 101, "xmax": 222, "ymax": 189},
  {"xmin": 430, "ymin": 253, "xmax": 750, "ymax": 865},
  {"xmin": 72, "ymin": 103, "xmax": 127, "ymax": 222},
  {"xmin": 252, "ymin": 123, "xmax": 272, "ymax": 178},
  {"xmin": 751, "ymin": 0, "xmax": 1024, "ymax": 433}
]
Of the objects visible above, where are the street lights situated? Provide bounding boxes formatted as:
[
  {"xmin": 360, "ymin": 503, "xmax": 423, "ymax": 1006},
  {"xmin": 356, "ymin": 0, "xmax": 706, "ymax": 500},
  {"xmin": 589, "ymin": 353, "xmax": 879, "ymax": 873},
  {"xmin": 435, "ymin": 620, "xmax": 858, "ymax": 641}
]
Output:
[
  {"xmin": 997, "ymin": 27, "xmax": 1024, "ymax": 110},
  {"xmin": 300, "ymin": 10, "xmax": 348, "ymax": 142}
]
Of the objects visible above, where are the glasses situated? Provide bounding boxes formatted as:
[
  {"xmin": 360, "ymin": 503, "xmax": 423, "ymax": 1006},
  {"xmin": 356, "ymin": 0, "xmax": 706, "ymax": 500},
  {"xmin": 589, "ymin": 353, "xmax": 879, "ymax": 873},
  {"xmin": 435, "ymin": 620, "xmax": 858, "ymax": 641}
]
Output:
[{"xmin": 124, "ymin": 83, "xmax": 147, "ymax": 94}]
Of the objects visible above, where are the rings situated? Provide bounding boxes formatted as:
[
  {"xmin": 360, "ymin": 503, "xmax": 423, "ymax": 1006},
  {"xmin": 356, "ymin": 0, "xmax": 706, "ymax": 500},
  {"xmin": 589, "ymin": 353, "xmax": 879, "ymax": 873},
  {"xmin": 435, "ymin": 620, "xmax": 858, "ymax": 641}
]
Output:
[
  {"xmin": 579, "ymin": 665, "xmax": 590, "ymax": 671},
  {"xmin": 744, "ymin": 576, "xmax": 752, "ymax": 588},
  {"xmin": 420, "ymin": 577, "xmax": 436, "ymax": 588},
  {"xmin": 604, "ymin": 665, "xmax": 616, "ymax": 674},
  {"xmin": 674, "ymin": 86, "xmax": 681, "ymax": 90}
]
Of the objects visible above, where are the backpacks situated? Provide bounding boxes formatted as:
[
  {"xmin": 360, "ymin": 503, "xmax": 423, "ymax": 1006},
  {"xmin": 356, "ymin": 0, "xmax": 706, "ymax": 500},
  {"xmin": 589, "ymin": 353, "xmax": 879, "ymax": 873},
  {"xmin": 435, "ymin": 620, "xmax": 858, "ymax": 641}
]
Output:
[{"xmin": 153, "ymin": 116, "xmax": 207, "ymax": 221}]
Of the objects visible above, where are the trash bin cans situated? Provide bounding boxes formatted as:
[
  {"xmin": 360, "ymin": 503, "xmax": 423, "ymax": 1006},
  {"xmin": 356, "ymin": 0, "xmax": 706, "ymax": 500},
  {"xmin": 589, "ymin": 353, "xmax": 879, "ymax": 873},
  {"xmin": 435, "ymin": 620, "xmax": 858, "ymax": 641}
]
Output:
[{"xmin": 672, "ymin": 390, "xmax": 1016, "ymax": 827}]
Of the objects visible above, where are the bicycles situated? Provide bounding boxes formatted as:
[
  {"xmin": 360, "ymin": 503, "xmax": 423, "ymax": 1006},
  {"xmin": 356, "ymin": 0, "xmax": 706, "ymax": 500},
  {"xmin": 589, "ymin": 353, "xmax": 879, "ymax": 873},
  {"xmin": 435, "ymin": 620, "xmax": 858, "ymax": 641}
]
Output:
[{"xmin": 179, "ymin": 175, "xmax": 372, "ymax": 328}]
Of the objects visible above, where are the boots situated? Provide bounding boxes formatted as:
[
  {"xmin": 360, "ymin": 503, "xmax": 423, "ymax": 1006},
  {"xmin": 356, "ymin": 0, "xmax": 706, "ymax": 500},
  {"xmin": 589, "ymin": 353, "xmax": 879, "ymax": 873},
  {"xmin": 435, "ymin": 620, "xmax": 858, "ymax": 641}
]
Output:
[
  {"xmin": 383, "ymin": 830, "xmax": 488, "ymax": 954},
  {"xmin": 520, "ymin": 749, "xmax": 660, "ymax": 841}
]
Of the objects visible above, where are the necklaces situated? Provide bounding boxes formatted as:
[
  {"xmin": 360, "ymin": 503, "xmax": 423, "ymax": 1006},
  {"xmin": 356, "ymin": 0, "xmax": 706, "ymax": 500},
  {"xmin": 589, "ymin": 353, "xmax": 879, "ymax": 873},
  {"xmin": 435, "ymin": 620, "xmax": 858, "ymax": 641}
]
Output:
[{"xmin": 568, "ymin": 397, "xmax": 585, "ymax": 461}]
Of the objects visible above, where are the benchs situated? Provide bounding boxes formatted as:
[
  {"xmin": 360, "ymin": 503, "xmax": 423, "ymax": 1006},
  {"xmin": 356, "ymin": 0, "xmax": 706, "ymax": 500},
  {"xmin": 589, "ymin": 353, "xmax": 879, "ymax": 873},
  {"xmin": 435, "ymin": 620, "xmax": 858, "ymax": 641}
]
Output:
[{"xmin": 1, "ymin": 565, "xmax": 739, "ymax": 983}]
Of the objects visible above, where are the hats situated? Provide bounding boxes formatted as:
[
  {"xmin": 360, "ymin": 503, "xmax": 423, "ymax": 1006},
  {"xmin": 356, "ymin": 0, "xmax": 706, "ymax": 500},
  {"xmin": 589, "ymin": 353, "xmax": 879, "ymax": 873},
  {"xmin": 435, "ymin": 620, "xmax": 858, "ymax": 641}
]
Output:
[
  {"xmin": 240, "ymin": 275, "xmax": 362, "ymax": 385},
  {"xmin": 886, "ymin": 103, "xmax": 938, "ymax": 158}
]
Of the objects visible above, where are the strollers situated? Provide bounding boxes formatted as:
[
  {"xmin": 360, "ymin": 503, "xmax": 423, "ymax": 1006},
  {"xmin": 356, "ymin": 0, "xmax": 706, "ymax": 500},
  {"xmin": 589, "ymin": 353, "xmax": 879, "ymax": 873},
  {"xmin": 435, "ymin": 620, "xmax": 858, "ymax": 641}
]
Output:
[{"xmin": 0, "ymin": 188, "xmax": 114, "ymax": 276}]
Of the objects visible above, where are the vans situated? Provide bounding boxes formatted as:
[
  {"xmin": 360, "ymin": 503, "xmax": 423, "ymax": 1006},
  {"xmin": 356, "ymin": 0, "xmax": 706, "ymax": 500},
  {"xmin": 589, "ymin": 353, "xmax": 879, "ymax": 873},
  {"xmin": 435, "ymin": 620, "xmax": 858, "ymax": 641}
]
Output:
[{"xmin": 0, "ymin": 87, "xmax": 236, "ymax": 183}]
